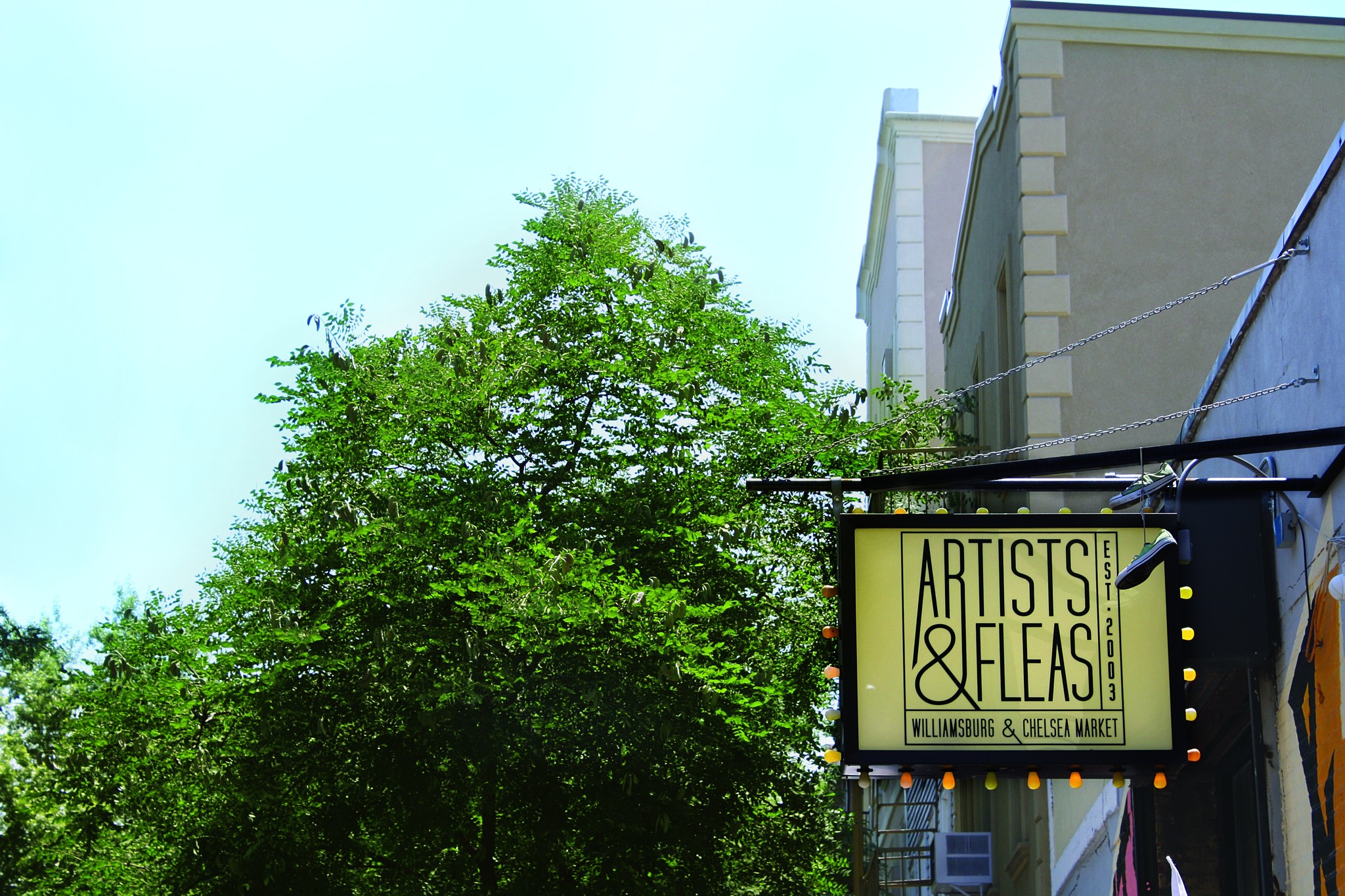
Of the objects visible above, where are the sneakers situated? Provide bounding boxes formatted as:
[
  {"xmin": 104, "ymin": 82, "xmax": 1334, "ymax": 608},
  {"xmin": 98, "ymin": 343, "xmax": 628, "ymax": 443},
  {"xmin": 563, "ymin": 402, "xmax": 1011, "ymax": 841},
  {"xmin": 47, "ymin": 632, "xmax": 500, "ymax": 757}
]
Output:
[
  {"xmin": 1109, "ymin": 462, "xmax": 1178, "ymax": 510},
  {"xmin": 1115, "ymin": 529, "xmax": 1178, "ymax": 590}
]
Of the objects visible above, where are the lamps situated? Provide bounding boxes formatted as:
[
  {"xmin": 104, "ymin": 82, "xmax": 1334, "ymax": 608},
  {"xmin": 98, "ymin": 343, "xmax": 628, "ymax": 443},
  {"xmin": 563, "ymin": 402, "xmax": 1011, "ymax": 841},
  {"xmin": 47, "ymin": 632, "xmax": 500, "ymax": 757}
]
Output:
[{"xmin": 822, "ymin": 491, "xmax": 1202, "ymax": 791}]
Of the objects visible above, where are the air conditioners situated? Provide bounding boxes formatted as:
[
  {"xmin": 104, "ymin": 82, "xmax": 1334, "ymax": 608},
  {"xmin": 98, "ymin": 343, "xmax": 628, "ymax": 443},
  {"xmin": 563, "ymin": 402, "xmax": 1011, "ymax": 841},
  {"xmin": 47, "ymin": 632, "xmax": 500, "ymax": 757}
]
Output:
[{"xmin": 928, "ymin": 831, "xmax": 992, "ymax": 893}]
